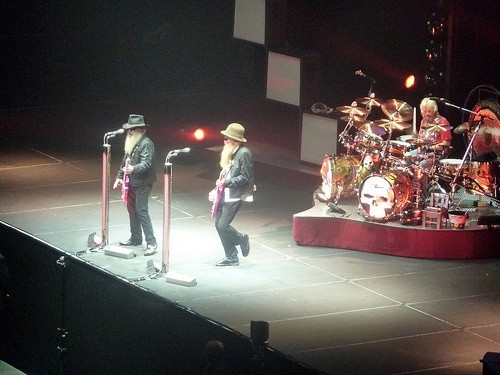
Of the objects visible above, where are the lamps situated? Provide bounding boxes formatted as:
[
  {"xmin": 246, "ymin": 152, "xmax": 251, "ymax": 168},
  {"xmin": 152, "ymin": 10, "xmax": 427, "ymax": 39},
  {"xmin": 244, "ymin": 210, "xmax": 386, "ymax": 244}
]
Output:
[
  {"xmin": 325, "ymin": 203, "xmax": 346, "ymax": 216},
  {"xmin": 476, "ymin": 215, "xmax": 500, "ymax": 228}
]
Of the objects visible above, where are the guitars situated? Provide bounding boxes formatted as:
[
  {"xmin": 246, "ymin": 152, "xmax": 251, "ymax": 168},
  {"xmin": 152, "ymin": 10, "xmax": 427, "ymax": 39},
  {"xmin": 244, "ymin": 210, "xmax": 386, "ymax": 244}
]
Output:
[
  {"xmin": 210, "ymin": 161, "xmax": 235, "ymax": 219},
  {"xmin": 120, "ymin": 155, "xmax": 130, "ymax": 204}
]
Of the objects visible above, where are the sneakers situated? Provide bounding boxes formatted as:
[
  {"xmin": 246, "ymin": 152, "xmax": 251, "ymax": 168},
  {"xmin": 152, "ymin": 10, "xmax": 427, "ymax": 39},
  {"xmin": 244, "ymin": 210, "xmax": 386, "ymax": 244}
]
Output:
[
  {"xmin": 119, "ymin": 239, "xmax": 142, "ymax": 245},
  {"xmin": 215, "ymin": 255, "xmax": 240, "ymax": 266},
  {"xmin": 144, "ymin": 242, "xmax": 158, "ymax": 255},
  {"xmin": 239, "ymin": 233, "xmax": 250, "ymax": 257}
]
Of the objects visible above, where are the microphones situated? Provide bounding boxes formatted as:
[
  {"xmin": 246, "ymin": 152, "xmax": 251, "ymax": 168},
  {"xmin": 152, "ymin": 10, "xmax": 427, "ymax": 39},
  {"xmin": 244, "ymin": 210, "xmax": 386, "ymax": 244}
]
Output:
[
  {"xmin": 107, "ymin": 129, "xmax": 124, "ymax": 135},
  {"xmin": 429, "ymin": 97, "xmax": 445, "ymax": 101},
  {"xmin": 172, "ymin": 148, "xmax": 190, "ymax": 153},
  {"xmin": 354, "ymin": 70, "xmax": 367, "ymax": 78}
]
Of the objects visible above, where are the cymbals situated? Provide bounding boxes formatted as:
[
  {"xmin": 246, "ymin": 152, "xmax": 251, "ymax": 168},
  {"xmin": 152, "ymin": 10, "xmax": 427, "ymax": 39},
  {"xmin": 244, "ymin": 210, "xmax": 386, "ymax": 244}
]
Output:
[
  {"xmin": 381, "ymin": 99, "xmax": 414, "ymax": 122},
  {"xmin": 356, "ymin": 97, "xmax": 391, "ymax": 105},
  {"xmin": 397, "ymin": 134, "xmax": 434, "ymax": 145},
  {"xmin": 334, "ymin": 105, "xmax": 374, "ymax": 115},
  {"xmin": 369, "ymin": 119, "xmax": 413, "ymax": 130},
  {"xmin": 421, "ymin": 123, "xmax": 453, "ymax": 131},
  {"xmin": 353, "ymin": 120, "xmax": 386, "ymax": 135},
  {"xmin": 341, "ymin": 115, "xmax": 366, "ymax": 122},
  {"xmin": 453, "ymin": 119, "xmax": 485, "ymax": 136}
]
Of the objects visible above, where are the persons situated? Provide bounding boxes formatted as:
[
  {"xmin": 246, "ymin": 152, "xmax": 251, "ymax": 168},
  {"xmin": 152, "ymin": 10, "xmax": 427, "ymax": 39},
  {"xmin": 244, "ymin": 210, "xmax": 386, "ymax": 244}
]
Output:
[
  {"xmin": 202, "ymin": 339, "xmax": 231, "ymax": 375},
  {"xmin": 400, "ymin": 96, "xmax": 451, "ymax": 205},
  {"xmin": 207, "ymin": 123, "xmax": 253, "ymax": 267},
  {"xmin": 112, "ymin": 114, "xmax": 156, "ymax": 256}
]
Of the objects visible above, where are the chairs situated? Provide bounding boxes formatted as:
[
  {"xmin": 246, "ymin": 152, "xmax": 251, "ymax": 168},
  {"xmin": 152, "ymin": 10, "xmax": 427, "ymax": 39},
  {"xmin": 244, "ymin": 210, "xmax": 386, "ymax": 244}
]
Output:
[{"xmin": 421, "ymin": 192, "xmax": 450, "ymax": 229}]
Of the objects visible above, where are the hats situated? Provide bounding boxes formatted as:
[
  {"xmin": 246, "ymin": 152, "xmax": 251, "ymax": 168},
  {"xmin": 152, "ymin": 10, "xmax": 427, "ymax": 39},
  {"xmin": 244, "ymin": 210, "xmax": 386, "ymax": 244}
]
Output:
[
  {"xmin": 221, "ymin": 123, "xmax": 247, "ymax": 143},
  {"xmin": 122, "ymin": 114, "xmax": 146, "ymax": 129}
]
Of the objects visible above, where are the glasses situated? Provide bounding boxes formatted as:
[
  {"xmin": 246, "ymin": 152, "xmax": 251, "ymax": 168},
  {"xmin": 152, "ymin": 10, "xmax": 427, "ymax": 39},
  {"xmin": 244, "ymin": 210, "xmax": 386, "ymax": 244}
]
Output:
[{"xmin": 223, "ymin": 138, "xmax": 229, "ymax": 141}]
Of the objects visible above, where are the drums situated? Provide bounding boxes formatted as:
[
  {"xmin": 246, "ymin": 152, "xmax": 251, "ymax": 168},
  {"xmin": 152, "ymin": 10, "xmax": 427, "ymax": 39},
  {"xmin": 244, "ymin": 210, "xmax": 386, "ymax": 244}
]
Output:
[
  {"xmin": 352, "ymin": 129, "xmax": 384, "ymax": 158},
  {"xmin": 321, "ymin": 152, "xmax": 362, "ymax": 200},
  {"xmin": 358, "ymin": 170, "xmax": 412, "ymax": 222},
  {"xmin": 381, "ymin": 141, "xmax": 411, "ymax": 164},
  {"xmin": 436, "ymin": 159, "xmax": 480, "ymax": 176}
]
